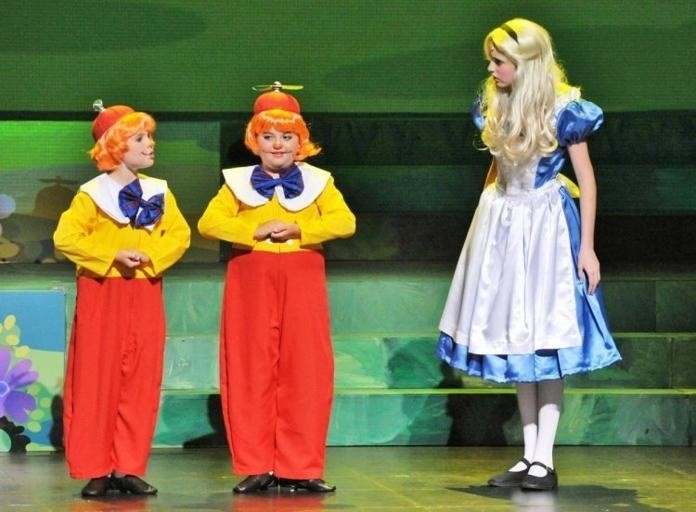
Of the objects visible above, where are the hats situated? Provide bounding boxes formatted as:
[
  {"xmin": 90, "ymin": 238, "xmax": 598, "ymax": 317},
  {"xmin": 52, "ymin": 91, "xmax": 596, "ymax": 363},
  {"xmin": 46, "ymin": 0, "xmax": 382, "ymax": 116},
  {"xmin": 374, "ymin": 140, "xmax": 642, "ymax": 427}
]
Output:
[
  {"xmin": 251, "ymin": 79, "xmax": 304, "ymax": 115},
  {"xmin": 91, "ymin": 98, "xmax": 135, "ymax": 142}
]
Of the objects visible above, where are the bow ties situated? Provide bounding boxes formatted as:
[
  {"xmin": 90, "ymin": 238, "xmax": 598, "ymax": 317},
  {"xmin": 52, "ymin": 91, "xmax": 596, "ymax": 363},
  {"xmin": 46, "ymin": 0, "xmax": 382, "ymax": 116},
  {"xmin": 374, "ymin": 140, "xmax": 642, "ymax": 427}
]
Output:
[
  {"xmin": 116, "ymin": 176, "xmax": 165, "ymax": 227},
  {"xmin": 250, "ymin": 163, "xmax": 304, "ymax": 200}
]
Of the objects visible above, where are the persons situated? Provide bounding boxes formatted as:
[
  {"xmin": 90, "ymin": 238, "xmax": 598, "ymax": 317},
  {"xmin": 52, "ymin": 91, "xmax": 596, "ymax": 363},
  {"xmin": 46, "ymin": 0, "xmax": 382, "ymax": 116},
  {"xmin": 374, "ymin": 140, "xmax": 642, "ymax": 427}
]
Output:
[
  {"xmin": 196, "ymin": 91, "xmax": 358, "ymax": 496},
  {"xmin": 435, "ymin": 17, "xmax": 624, "ymax": 492},
  {"xmin": 53, "ymin": 100, "xmax": 192, "ymax": 499}
]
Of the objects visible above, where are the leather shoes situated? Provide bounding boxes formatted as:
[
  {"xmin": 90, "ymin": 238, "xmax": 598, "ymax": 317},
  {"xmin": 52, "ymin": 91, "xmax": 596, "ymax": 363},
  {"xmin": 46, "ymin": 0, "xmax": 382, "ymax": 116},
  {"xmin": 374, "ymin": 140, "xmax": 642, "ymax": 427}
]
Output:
[
  {"xmin": 489, "ymin": 455, "xmax": 557, "ymax": 489},
  {"xmin": 82, "ymin": 471, "xmax": 158, "ymax": 496},
  {"xmin": 233, "ymin": 473, "xmax": 336, "ymax": 494}
]
